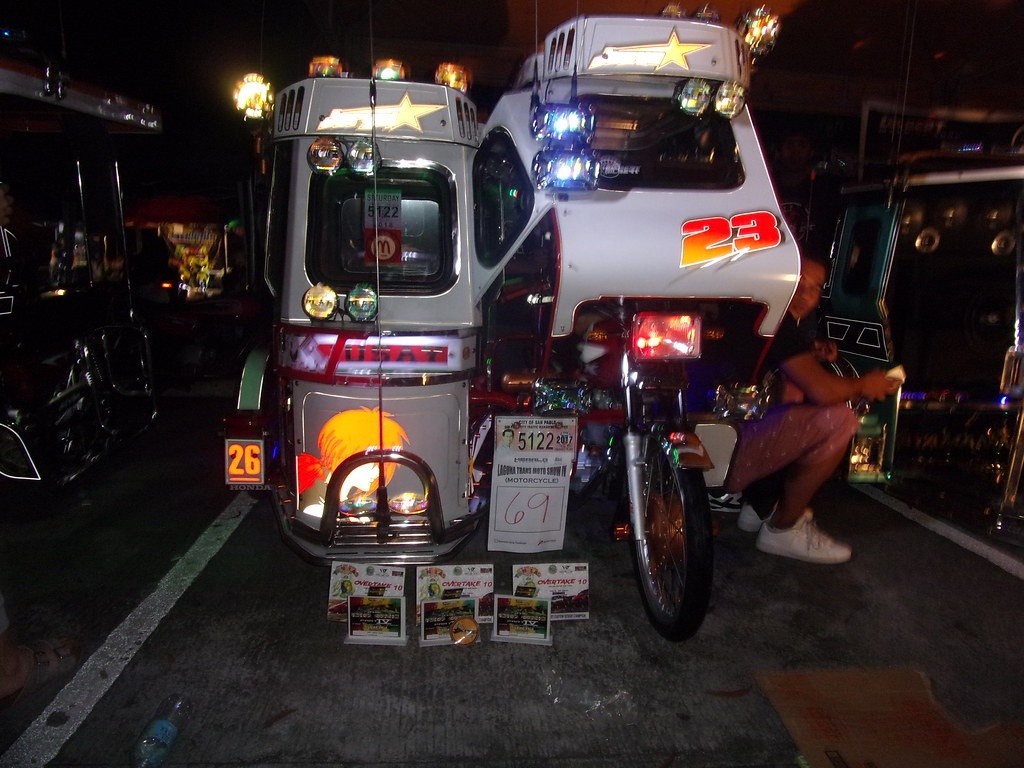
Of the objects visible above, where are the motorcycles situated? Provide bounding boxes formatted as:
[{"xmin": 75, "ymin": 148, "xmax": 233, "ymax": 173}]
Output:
[
  {"xmin": 224, "ymin": 14, "xmax": 803, "ymax": 643},
  {"xmin": 791, "ymin": 165, "xmax": 1024, "ymax": 581},
  {"xmin": 0, "ymin": 26, "xmax": 164, "ymax": 495}
]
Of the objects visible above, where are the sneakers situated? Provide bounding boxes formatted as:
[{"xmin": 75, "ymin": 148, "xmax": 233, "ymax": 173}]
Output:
[
  {"xmin": 755, "ymin": 515, "xmax": 851, "ymax": 564},
  {"xmin": 737, "ymin": 500, "xmax": 779, "ymax": 532},
  {"xmin": 708, "ymin": 492, "xmax": 742, "ymax": 512}
]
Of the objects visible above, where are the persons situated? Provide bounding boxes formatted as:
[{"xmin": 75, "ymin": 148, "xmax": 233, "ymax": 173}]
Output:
[
  {"xmin": 0, "ymin": 183, "xmax": 14, "ymax": 225},
  {"xmin": 622, "ymin": 79, "xmax": 739, "ymax": 187},
  {"xmin": 709, "ymin": 248, "xmax": 903, "ymax": 565}
]
